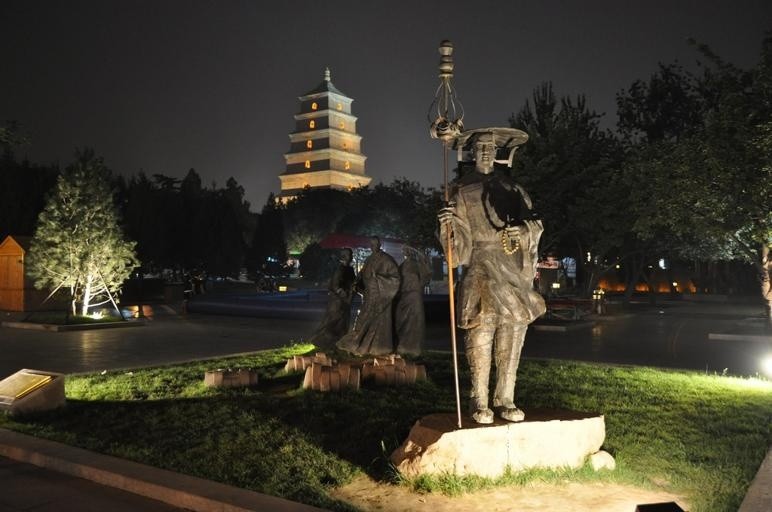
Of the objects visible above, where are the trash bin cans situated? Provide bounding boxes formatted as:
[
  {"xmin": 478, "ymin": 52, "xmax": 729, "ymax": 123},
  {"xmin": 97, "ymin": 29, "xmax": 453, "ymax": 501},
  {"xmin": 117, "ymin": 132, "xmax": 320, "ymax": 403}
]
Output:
[{"xmin": 163, "ymin": 281, "xmax": 185, "ymax": 315}]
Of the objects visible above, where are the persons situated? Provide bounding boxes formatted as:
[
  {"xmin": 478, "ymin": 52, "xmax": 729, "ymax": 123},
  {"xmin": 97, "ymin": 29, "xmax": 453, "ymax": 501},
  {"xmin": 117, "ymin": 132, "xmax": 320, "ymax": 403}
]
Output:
[
  {"xmin": 430, "ymin": 122, "xmax": 551, "ymax": 427},
  {"xmin": 332, "ymin": 234, "xmax": 402, "ymax": 362},
  {"xmin": 395, "ymin": 239, "xmax": 434, "ymax": 364},
  {"xmin": 299, "ymin": 245, "xmax": 358, "ymax": 347}
]
